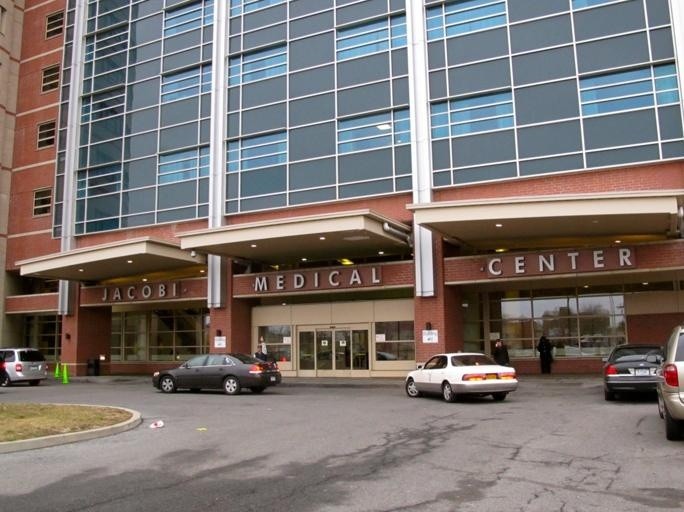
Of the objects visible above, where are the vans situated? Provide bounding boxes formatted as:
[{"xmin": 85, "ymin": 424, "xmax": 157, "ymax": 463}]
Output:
[{"xmin": 0, "ymin": 347, "xmax": 49, "ymax": 387}]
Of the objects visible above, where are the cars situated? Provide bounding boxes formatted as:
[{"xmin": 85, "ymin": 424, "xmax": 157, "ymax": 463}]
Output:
[
  {"xmin": 601, "ymin": 324, "xmax": 684, "ymax": 441},
  {"xmin": 152, "ymin": 354, "xmax": 281, "ymax": 395},
  {"xmin": 405, "ymin": 352, "xmax": 518, "ymax": 403},
  {"xmin": 377, "ymin": 352, "xmax": 396, "ymax": 360}
]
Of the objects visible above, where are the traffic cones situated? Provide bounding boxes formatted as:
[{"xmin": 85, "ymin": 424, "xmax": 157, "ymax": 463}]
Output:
[{"xmin": 54, "ymin": 362, "xmax": 69, "ymax": 384}]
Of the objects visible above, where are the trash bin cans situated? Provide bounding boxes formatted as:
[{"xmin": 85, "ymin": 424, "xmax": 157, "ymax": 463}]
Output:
[{"xmin": 87, "ymin": 358, "xmax": 100, "ymax": 376}]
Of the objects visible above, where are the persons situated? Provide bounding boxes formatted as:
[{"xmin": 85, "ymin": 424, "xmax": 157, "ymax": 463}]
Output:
[
  {"xmin": 254, "ymin": 345, "xmax": 267, "ymax": 361},
  {"xmin": 491, "ymin": 339, "xmax": 509, "ymax": 366},
  {"xmin": 537, "ymin": 336, "xmax": 552, "ymax": 374}
]
